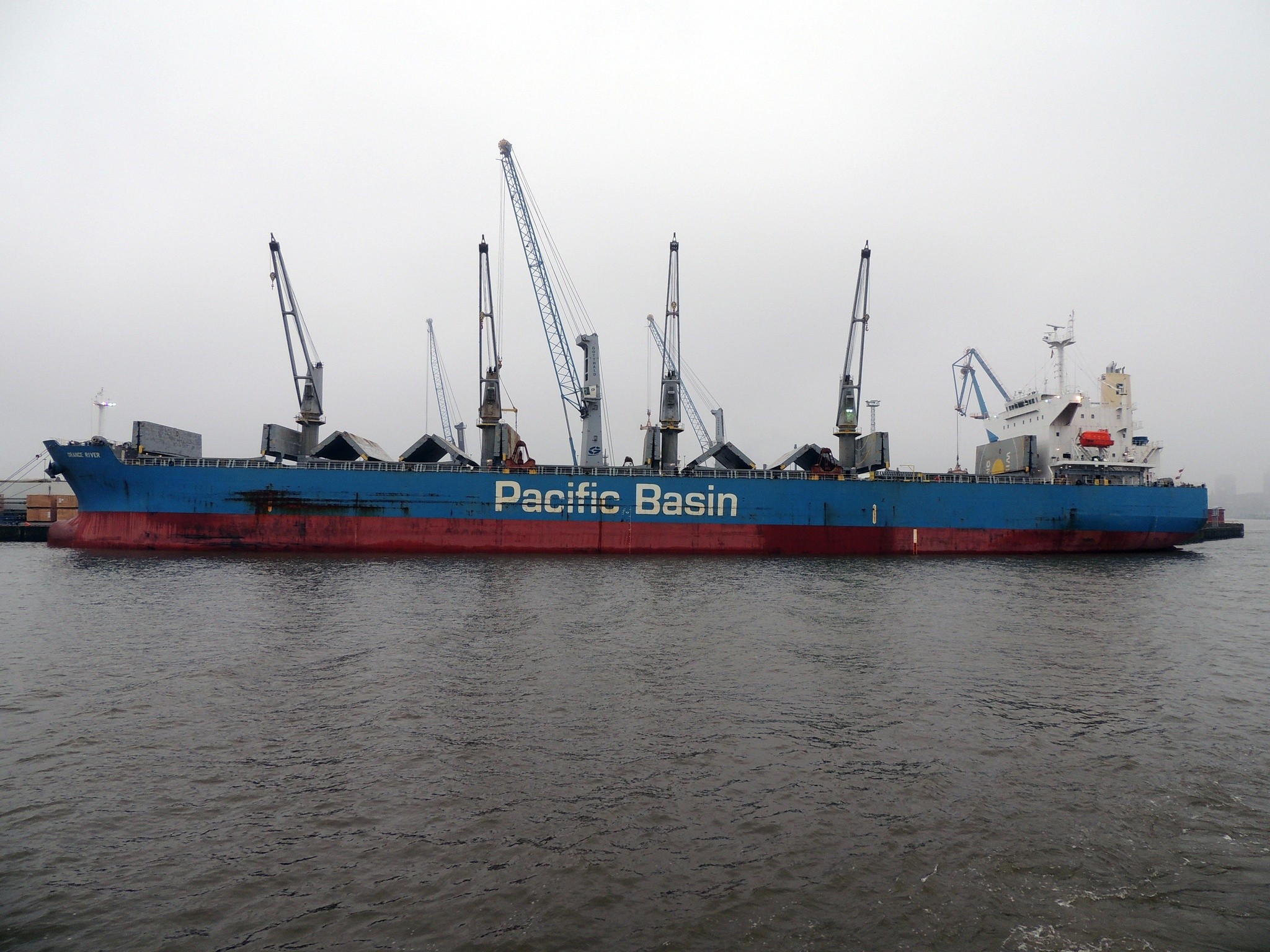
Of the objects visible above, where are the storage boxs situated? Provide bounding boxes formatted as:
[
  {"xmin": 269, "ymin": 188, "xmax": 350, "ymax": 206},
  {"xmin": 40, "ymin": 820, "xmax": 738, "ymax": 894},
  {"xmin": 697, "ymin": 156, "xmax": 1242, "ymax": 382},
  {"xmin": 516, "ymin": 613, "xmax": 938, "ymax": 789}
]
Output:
[{"xmin": 26, "ymin": 494, "xmax": 78, "ymax": 522}]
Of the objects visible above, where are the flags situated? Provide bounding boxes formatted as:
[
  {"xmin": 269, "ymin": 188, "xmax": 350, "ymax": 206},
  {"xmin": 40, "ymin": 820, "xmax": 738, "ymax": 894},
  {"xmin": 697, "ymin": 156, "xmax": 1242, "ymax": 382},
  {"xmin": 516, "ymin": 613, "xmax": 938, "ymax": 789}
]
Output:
[
  {"xmin": 1175, "ymin": 476, "xmax": 1180, "ymax": 479},
  {"xmin": 1178, "ymin": 469, "xmax": 1183, "ymax": 473}
]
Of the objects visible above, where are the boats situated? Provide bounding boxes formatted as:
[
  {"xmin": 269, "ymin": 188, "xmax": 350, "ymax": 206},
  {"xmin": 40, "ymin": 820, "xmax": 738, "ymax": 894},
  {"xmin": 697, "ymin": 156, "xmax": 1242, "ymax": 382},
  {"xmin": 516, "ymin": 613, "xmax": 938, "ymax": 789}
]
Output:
[
  {"xmin": 1079, "ymin": 430, "xmax": 1115, "ymax": 448},
  {"xmin": 42, "ymin": 138, "xmax": 1246, "ymax": 559}
]
